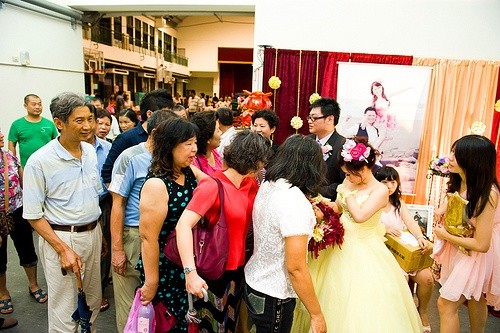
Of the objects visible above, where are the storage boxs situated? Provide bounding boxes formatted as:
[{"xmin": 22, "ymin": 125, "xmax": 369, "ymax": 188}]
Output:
[{"xmin": 383, "ymin": 229, "xmax": 436, "ymax": 272}]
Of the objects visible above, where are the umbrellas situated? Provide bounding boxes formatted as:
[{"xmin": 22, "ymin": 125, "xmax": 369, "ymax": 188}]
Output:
[
  {"xmin": 185, "ymin": 288, "xmax": 209, "ymax": 333},
  {"xmin": 61, "ymin": 265, "xmax": 92, "ymax": 333}
]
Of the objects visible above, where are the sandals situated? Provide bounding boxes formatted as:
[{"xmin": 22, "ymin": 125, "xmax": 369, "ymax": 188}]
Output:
[
  {"xmin": 0, "ymin": 295, "xmax": 13, "ymax": 314},
  {"xmin": 29, "ymin": 286, "xmax": 47, "ymax": 303}
]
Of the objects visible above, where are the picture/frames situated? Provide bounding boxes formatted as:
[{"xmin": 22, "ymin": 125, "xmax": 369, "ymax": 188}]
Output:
[{"xmin": 406, "ymin": 204, "xmax": 434, "ymax": 237}]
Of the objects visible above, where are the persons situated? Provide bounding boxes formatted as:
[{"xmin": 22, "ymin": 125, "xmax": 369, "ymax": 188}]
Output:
[
  {"xmin": 251, "ymin": 110, "xmax": 279, "ymax": 153},
  {"xmin": 435, "ymin": 135, "xmax": 500, "ymax": 333},
  {"xmin": 190, "ymin": 109, "xmax": 222, "ymax": 176},
  {"xmin": 289, "ymin": 136, "xmax": 424, "ymax": 333},
  {"xmin": 8, "ymin": 93, "xmax": 59, "ymax": 170},
  {"xmin": 345, "ymin": 81, "xmax": 410, "ymax": 156},
  {"xmin": 215, "ymin": 108, "xmax": 238, "ymax": 155},
  {"xmin": 307, "ymin": 98, "xmax": 347, "ymax": 201},
  {"xmin": 107, "ymin": 110, "xmax": 176, "ymax": 333},
  {"xmin": 23, "ymin": 93, "xmax": 105, "ymax": 333},
  {"xmin": 0, "ymin": 130, "xmax": 49, "ymax": 313},
  {"xmin": 100, "ymin": 89, "xmax": 172, "ymax": 184},
  {"xmin": 243, "ymin": 133, "xmax": 327, "ymax": 333},
  {"xmin": 176, "ymin": 130, "xmax": 270, "ymax": 333},
  {"xmin": 174, "ymin": 90, "xmax": 245, "ymax": 119},
  {"xmin": 377, "ymin": 166, "xmax": 435, "ymax": 333},
  {"xmin": 92, "ymin": 90, "xmax": 142, "ymax": 310},
  {"xmin": 138, "ymin": 117, "xmax": 201, "ymax": 333}
]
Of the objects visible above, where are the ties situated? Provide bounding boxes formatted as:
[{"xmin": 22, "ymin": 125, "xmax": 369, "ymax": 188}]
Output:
[{"xmin": 316, "ymin": 140, "xmax": 322, "ymax": 148}]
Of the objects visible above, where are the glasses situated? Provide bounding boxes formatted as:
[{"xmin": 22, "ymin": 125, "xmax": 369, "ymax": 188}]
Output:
[{"xmin": 306, "ymin": 114, "xmax": 330, "ymax": 122}]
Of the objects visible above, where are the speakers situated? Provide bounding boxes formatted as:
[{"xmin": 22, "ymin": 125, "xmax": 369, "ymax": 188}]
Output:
[{"xmin": 84, "ymin": 11, "xmax": 101, "ymax": 25}]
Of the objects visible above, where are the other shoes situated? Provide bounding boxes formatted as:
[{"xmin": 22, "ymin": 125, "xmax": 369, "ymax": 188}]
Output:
[
  {"xmin": 99, "ymin": 295, "xmax": 110, "ymax": 312},
  {"xmin": 422, "ymin": 324, "xmax": 431, "ymax": 333},
  {"xmin": 0, "ymin": 318, "xmax": 18, "ymax": 329}
]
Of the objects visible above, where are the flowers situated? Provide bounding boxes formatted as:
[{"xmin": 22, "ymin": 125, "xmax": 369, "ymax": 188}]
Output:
[
  {"xmin": 307, "ymin": 198, "xmax": 340, "ymax": 257},
  {"xmin": 291, "ymin": 117, "xmax": 304, "ymax": 129},
  {"xmin": 431, "ymin": 155, "xmax": 450, "ymax": 174},
  {"xmin": 309, "ymin": 92, "xmax": 322, "ymax": 103},
  {"xmin": 268, "ymin": 76, "xmax": 281, "ymax": 89},
  {"xmin": 322, "ymin": 143, "xmax": 333, "ymax": 161},
  {"xmin": 342, "ymin": 138, "xmax": 372, "ymax": 163}
]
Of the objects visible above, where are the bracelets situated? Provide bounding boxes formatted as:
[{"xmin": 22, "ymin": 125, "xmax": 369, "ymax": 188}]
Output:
[{"xmin": 417, "ymin": 236, "xmax": 427, "ymax": 240}]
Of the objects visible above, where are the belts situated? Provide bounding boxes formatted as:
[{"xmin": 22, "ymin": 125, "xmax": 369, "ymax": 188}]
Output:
[{"xmin": 49, "ymin": 215, "xmax": 102, "ymax": 233}]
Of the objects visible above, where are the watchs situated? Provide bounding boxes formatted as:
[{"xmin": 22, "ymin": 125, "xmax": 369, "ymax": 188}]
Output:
[{"xmin": 184, "ymin": 267, "xmax": 196, "ymax": 273}]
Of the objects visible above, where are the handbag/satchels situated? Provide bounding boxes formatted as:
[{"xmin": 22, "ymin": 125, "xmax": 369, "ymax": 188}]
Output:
[
  {"xmin": 163, "ymin": 177, "xmax": 228, "ymax": 280},
  {"xmin": 123, "ymin": 288, "xmax": 156, "ymax": 333}
]
